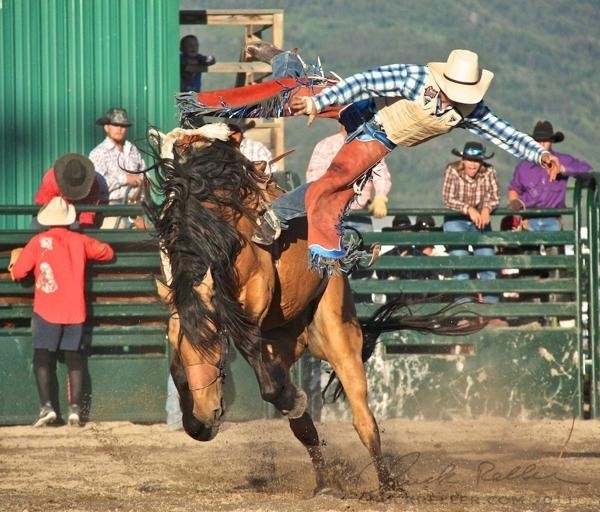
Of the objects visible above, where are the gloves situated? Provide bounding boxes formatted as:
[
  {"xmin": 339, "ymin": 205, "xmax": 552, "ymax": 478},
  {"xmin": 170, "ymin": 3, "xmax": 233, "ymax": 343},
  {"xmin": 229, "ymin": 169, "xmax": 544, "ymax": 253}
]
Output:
[
  {"xmin": 292, "ymin": 96, "xmax": 317, "ymax": 127},
  {"xmin": 8, "ymin": 247, "xmax": 24, "ymax": 272},
  {"xmin": 368, "ymin": 196, "xmax": 388, "ymax": 218},
  {"xmin": 541, "ymin": 153, "xmax": 561, "ymax": 183}
]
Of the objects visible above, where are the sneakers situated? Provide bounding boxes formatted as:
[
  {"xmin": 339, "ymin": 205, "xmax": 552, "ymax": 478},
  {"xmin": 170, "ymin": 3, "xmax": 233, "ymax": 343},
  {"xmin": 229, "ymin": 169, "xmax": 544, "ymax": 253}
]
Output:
[
  {"xmin": 68, "ymin": 404, "xmax": 81, "ymax": 426},
  {"xmin": 259, "ymin": 216, "xmax": 275, "ymax": 243},
  {"xmin": 32, "ymin": 401, "xmax": 58, "ymax": 427},
  {"xmin": 487, "ymin": 319, "xmax": 509, "ymax": 327},
  {"xmin": 457, "ymin": 320, "xmax": 470, "ymax": 328},
  {"xmin": 247, "ymin": 43, "xmax": 285, "ymax": 65}
]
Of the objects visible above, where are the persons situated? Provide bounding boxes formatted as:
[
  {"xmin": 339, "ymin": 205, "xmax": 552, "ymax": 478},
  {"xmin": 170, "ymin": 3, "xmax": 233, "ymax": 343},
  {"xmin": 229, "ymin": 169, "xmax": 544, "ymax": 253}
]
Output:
[
  {"xmin": 172, "ymin": 38, "xmax": 563, "ymax": 282},
  {"xmin": 88, "ymin": 109, "xmax": 148, "ymax": 229},
  {"xmin": 32, "ymin": 155, "xmax": 110, "ymax": 230},
  {"xmin": 224, "ymin": 114, "xmax": 592, "ymax": 329},
  {"xmin": 5, "ymin": 196, "xmax": 114, "ymax": 426},
  {"xmin": 158, "ymin": 235, "xmax": 183, "ymax": 432},
  {"xmin": 179, "ymin": 34, "xmax": 216, "ymax": 128}
]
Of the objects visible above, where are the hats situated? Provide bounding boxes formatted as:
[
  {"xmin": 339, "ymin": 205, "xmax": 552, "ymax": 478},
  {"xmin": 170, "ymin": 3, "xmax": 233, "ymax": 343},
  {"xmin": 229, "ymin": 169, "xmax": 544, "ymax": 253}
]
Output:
[
  {"xmin": 427, "ymin": 49, "xmax": 495, "ymax": 104},
  {"xmin": 382, "ymin": 215, "xmax": 443, "ymax": 231},
  {"xmin": 96, "ymin": 108, "xmax": 133, "ymax": 127},
  {"xmin": 224, "ymin": 118, "xmax": 255, "ymax": 133},
  {"xmin": 37, "ymin": 196, "xmax": 77, "ymax": 226},
  {"xmin": 452, "ymin": 142, "xmax": 495, "ymax": 160},
  {"xmin": 54, "ymin": 153, "xmax": 95, "ymax": 200},
  {"xmin": 501, "ymin": 216, "xmax": 519, "ymax": 228},
  {"xmin": 529, "ymin": 121, "xmax": 564, "ymax": 142}
]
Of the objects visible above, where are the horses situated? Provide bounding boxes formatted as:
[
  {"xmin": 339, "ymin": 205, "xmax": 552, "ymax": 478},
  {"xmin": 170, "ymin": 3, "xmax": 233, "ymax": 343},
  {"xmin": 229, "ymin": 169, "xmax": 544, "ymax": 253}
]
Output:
[{"xmin": 117, "ymin": 122, "xmax": 495, "ymax": 500}]
